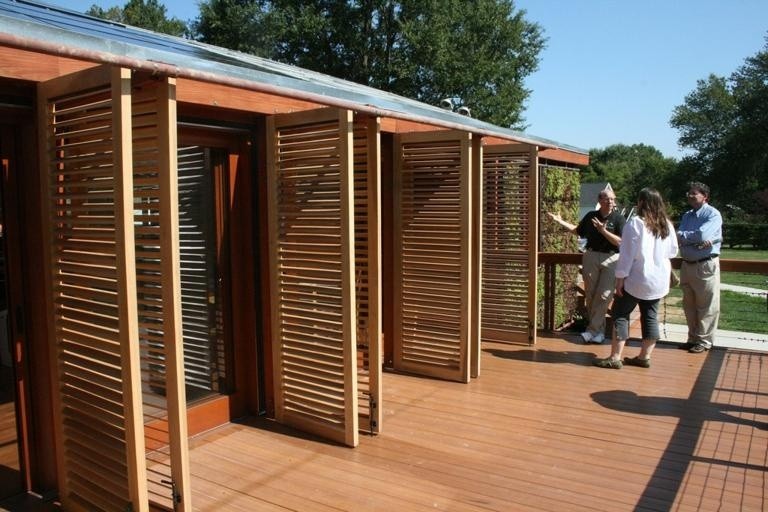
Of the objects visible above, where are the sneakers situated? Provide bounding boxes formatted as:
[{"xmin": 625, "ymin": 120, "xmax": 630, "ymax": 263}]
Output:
[
  {"xmin": 576, "ymin": 332, "xmax": 605, "ymax": 345},
  {"xmin": 678, "ymin": 342, "xmax": 706, "ymax": 353},
  {"xmin": 624, "ymin": 356, "xmax": 650, "ymax": 368},
  {"xmin": 592, "ymin": 358, "xmax": 622, "ymax": 369}
]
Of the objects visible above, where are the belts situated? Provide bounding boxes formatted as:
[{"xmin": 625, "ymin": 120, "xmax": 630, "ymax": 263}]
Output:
[
  {"xmin": 584, "ymin": 246, "xmax": 619, "ymax": 254},
  {"xmin": 683, "ymin": 254, "xmax": 718, "ymax": 264}
]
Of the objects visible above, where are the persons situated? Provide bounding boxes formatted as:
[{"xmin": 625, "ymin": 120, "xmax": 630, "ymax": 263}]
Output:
[
  {"xmin": 545, "ymin": 190, "xmax": 626, "ymax": 344},
  {"xmin": 673, "ymin": 181, "xmax": 723, "ymax": 353},
  {"xmin": 591, "ymin": 186, "xmax": 679, "ymax": 370}
]
